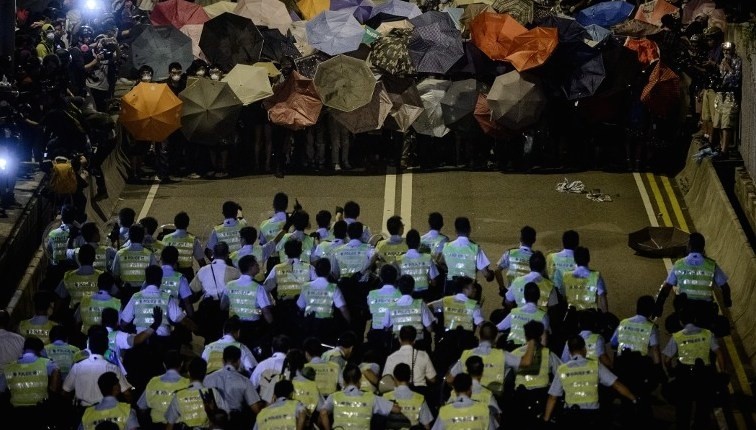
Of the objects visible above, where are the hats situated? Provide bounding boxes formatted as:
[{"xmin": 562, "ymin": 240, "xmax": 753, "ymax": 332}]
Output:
[{"xmin": 42, "ymin": 23, "xmax": 55, "ymax": 32}]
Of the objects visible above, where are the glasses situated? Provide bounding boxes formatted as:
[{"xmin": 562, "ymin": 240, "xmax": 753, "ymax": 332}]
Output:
[
  {"xmin": 169, "ymin": 71, "xmax": 182, "ymax": 75},
  {"xmin": 210, "ymin": 70, "xmax": 222, "ymax": 73},
  {"xmin": 141, "ymin": 71, "xmax": 151, "ymax": 76}
]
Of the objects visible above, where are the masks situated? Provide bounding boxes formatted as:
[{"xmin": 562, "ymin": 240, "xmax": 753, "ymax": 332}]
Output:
[
  {"xmin": 210, "ymin": 75, "xmax": 220, "ymax": 81},
  {"xmin": 195, "ymin": 71, "xmax": 205, "ymax": 76},
  {"xmin": 139, "ymin": 76, "xmax": 152, "ymax": 82},
  {"xmin": 44, "ymin": 33, "xmax": 54, "ymax": 39},
  {"xmin": 170, "ymin": 75, "xmax": 181, "ymax": 81}
]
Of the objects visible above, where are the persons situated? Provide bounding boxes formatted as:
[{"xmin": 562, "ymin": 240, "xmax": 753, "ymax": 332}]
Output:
[
  {"xmin": 130, "ymin": 59, "xmax": 657, "ymax": 184},
  {"xmin": 0, "ymin": 191, "xmax": 736, "ymax": 430},
  {"xmin": 624, "ymin": 12, "xmax": 743, "ymax": 152},
  {"xmin": 0, "ymin": 0, "xmax": 131, "ymax": 216}
]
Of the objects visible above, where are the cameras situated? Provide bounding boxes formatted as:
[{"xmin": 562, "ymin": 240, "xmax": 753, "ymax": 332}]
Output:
[
  {"xmin": 676, "ymin": 56, "xmax": 689, "ymax": 71},
  {"xmin": 85, "ymin": 70, "xmax": 100, "ymax": 83}
]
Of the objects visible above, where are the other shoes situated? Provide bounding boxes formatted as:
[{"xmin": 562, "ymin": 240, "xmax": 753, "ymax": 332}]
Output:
[
  {"xmin": 93, "ymin": 192, "xmax": 108, "ymax": 201},
  {"xmin": 135, "ymin": 153, "xmax": 669, "ymax": 185},
  {"xmin": 685, "ymin": 110, "xmax": 725, "ymax": 159}
]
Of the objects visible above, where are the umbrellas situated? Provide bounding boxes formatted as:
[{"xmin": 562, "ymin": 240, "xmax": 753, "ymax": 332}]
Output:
[
  {"xmin": 118, "ymin": 0, "xmax": 726, "ymax": 141},
  {"xmin": 627, "ymin": 212, "xmax": 691, "ymax": 258}
]
